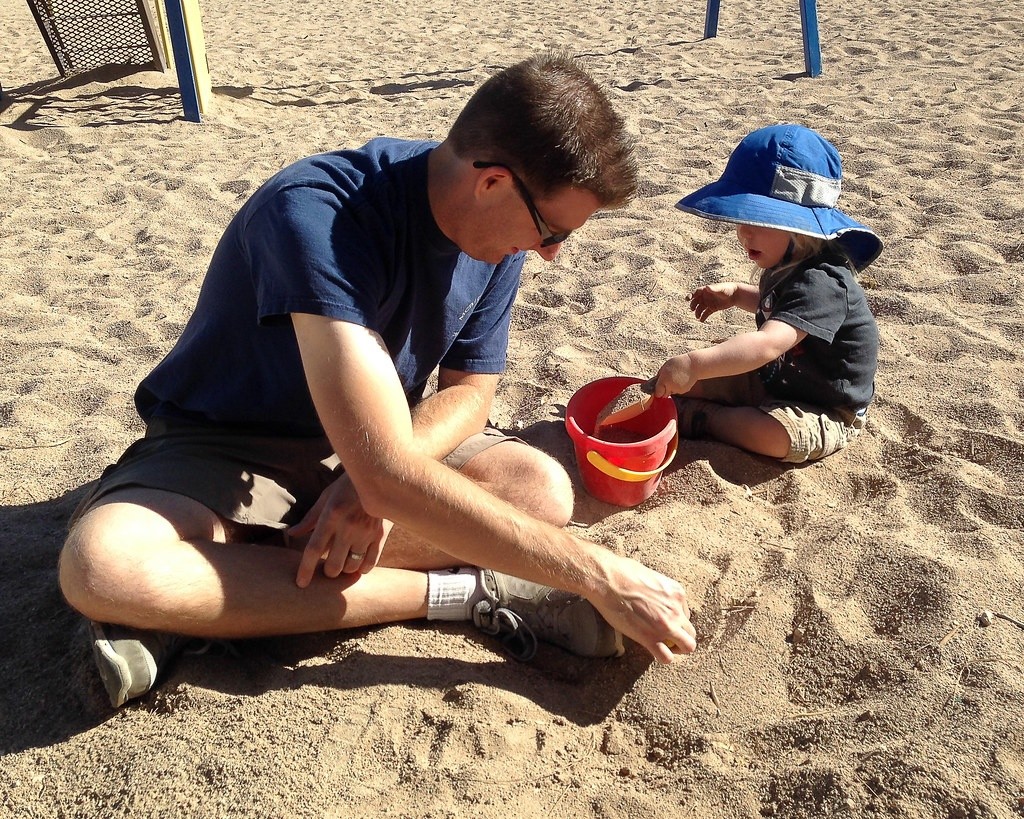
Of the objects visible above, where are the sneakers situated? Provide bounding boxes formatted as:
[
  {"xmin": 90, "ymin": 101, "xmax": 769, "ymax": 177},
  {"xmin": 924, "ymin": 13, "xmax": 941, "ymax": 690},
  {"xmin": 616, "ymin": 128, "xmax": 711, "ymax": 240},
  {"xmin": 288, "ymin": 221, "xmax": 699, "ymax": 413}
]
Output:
[
  {"xmin": 470, "ymin": 564, "xmax": 633, "ymax": 655},
  {"xmin": 89, "ymin": 621, "xmax": 159, "ymax": 707}
]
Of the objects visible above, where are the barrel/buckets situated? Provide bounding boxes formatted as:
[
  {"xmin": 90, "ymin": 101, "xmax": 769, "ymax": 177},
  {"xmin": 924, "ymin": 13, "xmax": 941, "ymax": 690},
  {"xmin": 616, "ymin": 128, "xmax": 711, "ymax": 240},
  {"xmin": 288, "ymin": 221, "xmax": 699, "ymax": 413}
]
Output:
[{"xmin": 565, "ymin": 376, "xmax": 678, "ymax": 507}]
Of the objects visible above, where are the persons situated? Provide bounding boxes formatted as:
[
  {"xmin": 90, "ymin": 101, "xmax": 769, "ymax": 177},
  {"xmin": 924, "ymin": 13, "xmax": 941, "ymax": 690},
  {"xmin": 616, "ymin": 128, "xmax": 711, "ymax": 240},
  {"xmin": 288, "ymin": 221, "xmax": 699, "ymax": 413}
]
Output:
[
  {"xmin": 57, "ymin": 54, "xmax": 697, "ymax": 710},
  {"xmin": 653, "ymin": 125, "xmax": 883, "ymax": 464}
]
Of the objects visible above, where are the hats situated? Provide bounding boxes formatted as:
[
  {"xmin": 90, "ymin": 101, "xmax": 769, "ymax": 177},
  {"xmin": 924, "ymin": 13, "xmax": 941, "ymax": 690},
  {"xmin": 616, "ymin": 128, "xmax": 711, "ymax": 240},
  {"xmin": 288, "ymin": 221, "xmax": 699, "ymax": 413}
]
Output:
[{"xmin": 674, "ymin": 125, "xmax": 882, "ymax": 276}]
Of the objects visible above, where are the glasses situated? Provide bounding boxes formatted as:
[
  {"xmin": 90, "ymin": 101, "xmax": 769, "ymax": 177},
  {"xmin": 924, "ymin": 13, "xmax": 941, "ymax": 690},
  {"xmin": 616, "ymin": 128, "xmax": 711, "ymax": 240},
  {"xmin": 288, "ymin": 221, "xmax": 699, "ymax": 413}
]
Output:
[{"xmin": 472, "ymin": 159, "xmax": 568, "ymax": 248}]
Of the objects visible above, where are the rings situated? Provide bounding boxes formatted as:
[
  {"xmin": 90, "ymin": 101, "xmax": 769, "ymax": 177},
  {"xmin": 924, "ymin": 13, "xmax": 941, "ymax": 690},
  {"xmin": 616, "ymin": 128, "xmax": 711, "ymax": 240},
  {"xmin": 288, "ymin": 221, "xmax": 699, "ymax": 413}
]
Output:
[{"xmin": 348, "ymin": 551, "xmax": 366, "ymax": 561}]
